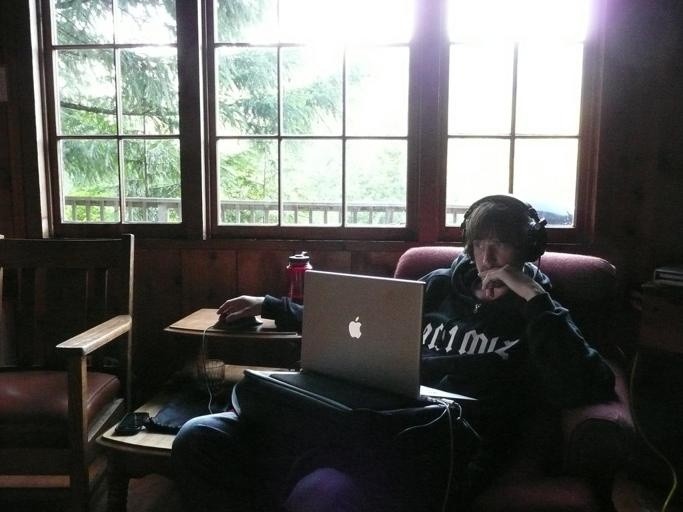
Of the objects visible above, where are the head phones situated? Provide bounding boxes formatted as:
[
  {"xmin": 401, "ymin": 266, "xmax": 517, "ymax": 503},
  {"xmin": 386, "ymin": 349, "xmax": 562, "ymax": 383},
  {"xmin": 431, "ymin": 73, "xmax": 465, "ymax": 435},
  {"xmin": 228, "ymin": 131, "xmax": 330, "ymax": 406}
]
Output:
[{"xmin": 462, "ymin": 194, "xmax": 549, "ymax": 261}]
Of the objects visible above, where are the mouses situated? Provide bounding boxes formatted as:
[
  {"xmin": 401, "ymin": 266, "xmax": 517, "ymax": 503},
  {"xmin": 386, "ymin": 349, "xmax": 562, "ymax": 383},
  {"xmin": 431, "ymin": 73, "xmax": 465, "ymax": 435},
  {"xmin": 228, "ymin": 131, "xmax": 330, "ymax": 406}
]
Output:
[{"xmin": 214, "ymin": 315, "xmax": 257, "ymax": 328}]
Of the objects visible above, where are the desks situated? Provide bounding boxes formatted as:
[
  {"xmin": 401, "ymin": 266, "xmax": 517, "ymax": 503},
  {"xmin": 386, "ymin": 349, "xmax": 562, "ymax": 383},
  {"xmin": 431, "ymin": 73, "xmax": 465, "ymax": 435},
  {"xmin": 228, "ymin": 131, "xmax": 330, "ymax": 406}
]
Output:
[{"xmin": 93, "ymin": 307, "xmax": 302, "ymax": 512}]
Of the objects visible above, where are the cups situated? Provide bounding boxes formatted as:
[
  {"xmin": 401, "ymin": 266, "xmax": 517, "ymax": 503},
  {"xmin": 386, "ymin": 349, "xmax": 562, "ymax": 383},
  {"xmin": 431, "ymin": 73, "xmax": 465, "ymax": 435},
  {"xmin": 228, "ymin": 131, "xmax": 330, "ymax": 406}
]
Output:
[{"xmin": 200, "ymin": 358, "xmax": 225, "ymax": 398}]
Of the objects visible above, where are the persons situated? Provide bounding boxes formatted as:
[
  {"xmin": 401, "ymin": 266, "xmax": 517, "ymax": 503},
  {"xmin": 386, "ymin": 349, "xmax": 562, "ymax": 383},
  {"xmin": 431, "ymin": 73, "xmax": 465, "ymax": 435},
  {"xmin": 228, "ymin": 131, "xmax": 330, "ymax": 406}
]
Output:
[{"xmin": 168, "ymin": 194, "xmax": 615, "ymax": 512}]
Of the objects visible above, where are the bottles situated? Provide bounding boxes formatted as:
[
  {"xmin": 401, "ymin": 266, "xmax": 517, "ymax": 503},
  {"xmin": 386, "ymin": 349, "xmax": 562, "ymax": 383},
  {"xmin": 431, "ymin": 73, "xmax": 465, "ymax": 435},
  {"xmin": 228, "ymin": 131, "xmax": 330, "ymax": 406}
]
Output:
[{"xmin": 284, "ymin": 249, "xmax": 312, "ymax": 305}]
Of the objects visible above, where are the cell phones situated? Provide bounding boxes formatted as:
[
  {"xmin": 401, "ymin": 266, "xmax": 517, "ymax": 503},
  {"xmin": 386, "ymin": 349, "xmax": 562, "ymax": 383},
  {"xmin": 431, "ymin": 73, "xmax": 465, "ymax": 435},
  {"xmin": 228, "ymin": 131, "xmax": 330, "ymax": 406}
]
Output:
[{"xmin": 114, "ymin": 412, "xmax": 149, "ymax": 435}]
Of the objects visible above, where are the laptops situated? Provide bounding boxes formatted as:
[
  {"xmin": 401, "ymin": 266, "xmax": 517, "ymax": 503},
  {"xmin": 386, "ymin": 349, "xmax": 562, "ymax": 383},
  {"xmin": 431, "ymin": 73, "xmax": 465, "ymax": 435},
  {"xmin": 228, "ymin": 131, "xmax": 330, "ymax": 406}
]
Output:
[{"xmin": 300, "ymin": 268, "xmax": 481, "ymax": 405}]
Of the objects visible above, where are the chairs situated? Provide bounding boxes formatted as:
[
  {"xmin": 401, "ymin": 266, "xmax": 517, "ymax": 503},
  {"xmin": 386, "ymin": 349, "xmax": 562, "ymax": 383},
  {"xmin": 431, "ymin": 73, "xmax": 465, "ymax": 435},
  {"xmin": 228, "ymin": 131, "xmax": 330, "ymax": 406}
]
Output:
[
  {"xmin": 0, "ymin": 234, "xmax": 135, "ymax": 512},
  {"xmin": 394, "ymin": 246, "xmax": 638, "ymax": 512}
]
